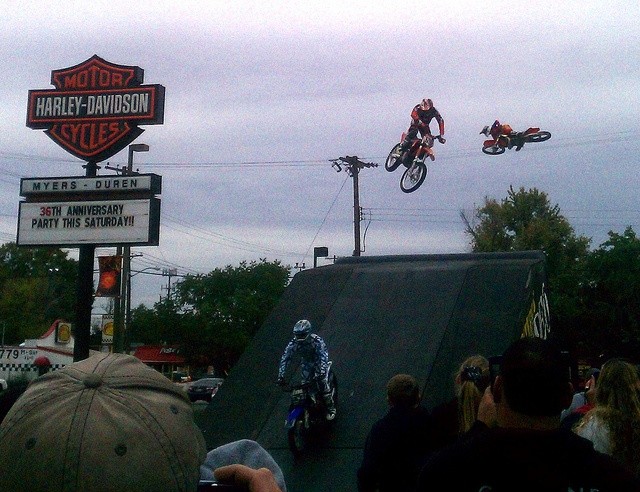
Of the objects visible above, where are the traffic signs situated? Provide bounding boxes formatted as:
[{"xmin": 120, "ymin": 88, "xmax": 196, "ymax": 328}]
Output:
[
  {"xmin": 20, "ymin": 174, "xmax": 151, "ymax": 194},
  {"xmin": 18, "ymin": 202, "xmax": 150, "ymax": 245}
]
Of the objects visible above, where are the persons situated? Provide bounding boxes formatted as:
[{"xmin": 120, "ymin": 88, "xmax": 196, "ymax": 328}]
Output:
[
  {"xmin": 417, "ymin": 337, "xmax": 640, "ymax": 490},
  {"xmin": 0, "ymin": 353, "xmax": 287, "ymax": 492},
  {"xmin": 355, "ymin": 375, "xmax": 435, "ymax": 490},
  {"xmin": 572, "ymin": 358, "xmax": 640, "ymax": 473},
  {"xmin": 276, "ymin": 319, "xmax": 338, "ymax": 422},
  {"xmin": 479, "ymin": 120, "xmax": 523, "ymax": 151},
  {"xmin": 559, "ymin": 368, "xmax": 604, "ymax": 429},
  {"xmin": 398, "ymin": 98, "xmax": 445, "ymax": 158},
  {"xmin": 452, "ymin": 355, "xmax": 494, "ymax": 432}
]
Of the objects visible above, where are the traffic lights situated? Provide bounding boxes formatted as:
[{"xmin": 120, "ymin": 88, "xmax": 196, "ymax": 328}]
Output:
[{"xmin": 96, "ymin": 258, "xmax": 120, "ymax": 298}]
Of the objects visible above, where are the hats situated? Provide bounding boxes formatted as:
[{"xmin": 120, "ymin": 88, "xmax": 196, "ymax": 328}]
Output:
[{"xmin": 2, "ymin": 353, "xmax": 208, "ymax": 489}]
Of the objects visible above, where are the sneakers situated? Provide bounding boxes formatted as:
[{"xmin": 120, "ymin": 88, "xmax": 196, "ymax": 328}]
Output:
[{"xmin": 325, "ymin": 409, "xmax": 337, "ymax": 420}]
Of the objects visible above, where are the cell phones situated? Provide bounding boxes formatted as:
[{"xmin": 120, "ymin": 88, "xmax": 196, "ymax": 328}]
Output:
[{"xmin": 489, "ymin": 354, "xmax": 504, "ymax": 400}]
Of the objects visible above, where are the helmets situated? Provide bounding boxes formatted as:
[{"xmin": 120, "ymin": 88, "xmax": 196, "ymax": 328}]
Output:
[
  {"xmin": 480, "ymin": 125, "xmax": 491, "ymax": 137},
  {"xmin": 293, "ymin": 319, "xmax": 312, "ymax": 342},
  {"xmin": 419, "ymin": 98, "xmax": 433, "ymax": 112}
]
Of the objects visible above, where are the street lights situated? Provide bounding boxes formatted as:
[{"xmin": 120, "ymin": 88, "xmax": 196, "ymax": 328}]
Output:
[
  {"xmin": 118, "ymin": 143, "xmax": 149, "ymax": 346},
  {"xmin": 126, "ymin": 267, "xmax": 161, "ymax": 356}
]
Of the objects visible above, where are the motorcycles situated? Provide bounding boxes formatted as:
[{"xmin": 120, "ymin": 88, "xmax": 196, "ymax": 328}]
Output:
[
  {"xmin": 281, "ymin": 360, "xmax": 337, "ymax": 455},
  {"xmin": 480, "ymin": 119, "xmax": 551, "ymax": 154},
  {"xmin": 385, "ymin": 132, "xmax": 446, "ymax": 193}
]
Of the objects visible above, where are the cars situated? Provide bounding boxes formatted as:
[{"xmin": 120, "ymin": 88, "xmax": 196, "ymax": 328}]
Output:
[{"xmin": 190, "ymin": 378, "xmax": 223, "ymax": 401}]
